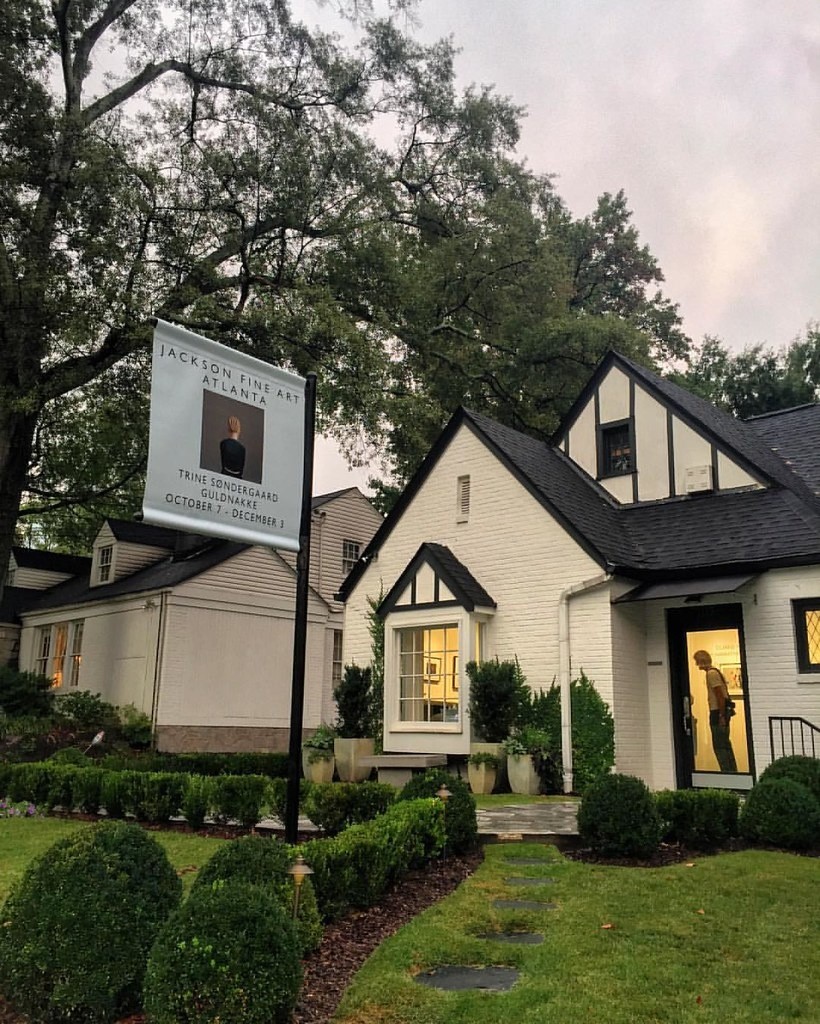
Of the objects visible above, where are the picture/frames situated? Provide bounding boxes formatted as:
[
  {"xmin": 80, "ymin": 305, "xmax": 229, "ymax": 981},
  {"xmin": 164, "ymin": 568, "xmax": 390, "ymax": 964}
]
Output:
[
  {"xmin": 423, "ymin": 656, "xmax": 441, "ymax": 682},
  {"xmin": 452, "ymin": 655, "xmax": 458, "ymax": 689},
  {"xmin": 719, "ymin": 663, "xmax": 744, "ymax": 695}
]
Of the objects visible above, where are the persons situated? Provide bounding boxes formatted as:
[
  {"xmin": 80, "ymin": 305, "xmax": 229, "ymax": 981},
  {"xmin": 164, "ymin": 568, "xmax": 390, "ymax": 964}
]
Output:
[
  {"xmin": 220, "ymin": 418, "xmax": 245, "ymax": 479},
  {"xmin": 693, "ymin": 651, "xmax": 737, "ymax": 771}
]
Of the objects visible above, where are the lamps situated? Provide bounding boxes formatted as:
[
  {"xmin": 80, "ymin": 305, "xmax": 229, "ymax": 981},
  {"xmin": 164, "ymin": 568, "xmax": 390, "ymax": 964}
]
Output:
[
  {"xmin": 684, "ymin": 595, "xmax": 704, "ymax": 604},
  {"xmin": 360, "ymin": 552, "xmax": 378, "ymax": 563}
]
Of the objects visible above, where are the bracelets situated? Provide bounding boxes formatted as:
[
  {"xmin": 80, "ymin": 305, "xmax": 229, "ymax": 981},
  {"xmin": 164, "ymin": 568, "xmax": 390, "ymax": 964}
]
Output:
[{"xmin": 719, "ymin": 714, "xmax": 725, "ymax": 716}]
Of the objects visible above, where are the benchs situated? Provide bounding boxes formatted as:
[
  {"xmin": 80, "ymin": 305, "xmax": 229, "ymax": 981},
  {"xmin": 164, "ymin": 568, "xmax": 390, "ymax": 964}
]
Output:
[{"xmin": 356, "ymin": 755, "xmax": 447, "ymax": 786}]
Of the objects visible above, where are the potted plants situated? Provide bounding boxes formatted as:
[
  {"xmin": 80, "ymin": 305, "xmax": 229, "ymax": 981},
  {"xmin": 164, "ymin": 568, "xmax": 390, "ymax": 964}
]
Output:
[
  {"xmin": 465, "ymin": 659, "xmax": 553, "ymax": 795},
  {"xmin": 332, "ymin": 655, "xmax": 376, "ymax": 782},
  {"xmin": 302, "ymin": 722, "xmax": 335, "ymax": 783}
]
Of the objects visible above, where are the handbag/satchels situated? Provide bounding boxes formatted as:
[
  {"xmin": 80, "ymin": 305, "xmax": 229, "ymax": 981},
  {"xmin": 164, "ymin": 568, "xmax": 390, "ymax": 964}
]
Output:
[{"xmin": 725, "ymin": 697, "xmax": 736, "ymax": 717}]
